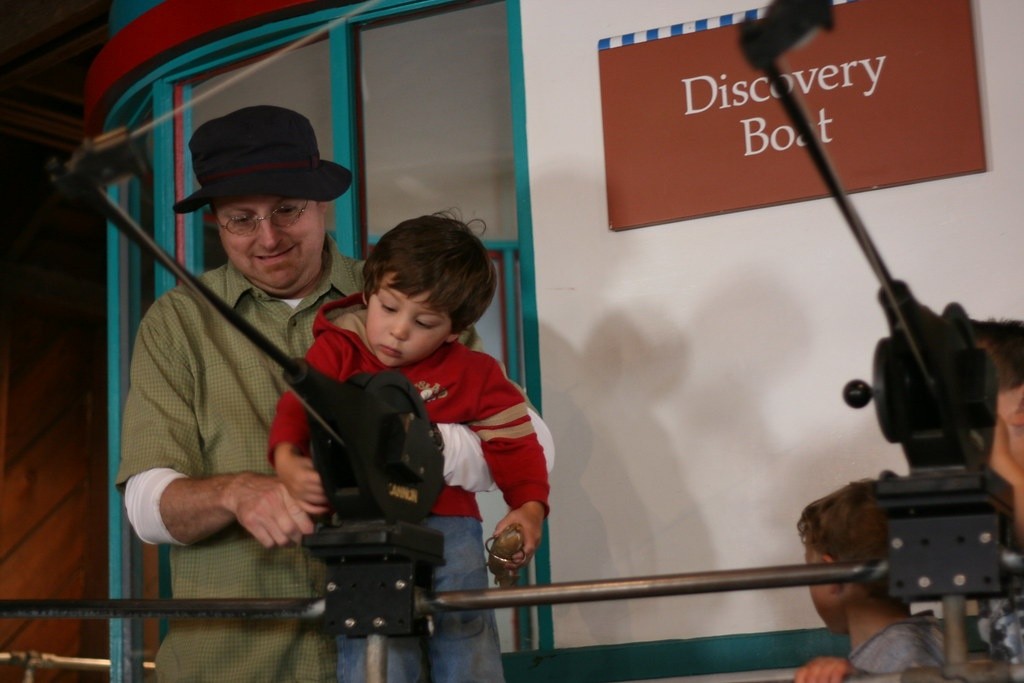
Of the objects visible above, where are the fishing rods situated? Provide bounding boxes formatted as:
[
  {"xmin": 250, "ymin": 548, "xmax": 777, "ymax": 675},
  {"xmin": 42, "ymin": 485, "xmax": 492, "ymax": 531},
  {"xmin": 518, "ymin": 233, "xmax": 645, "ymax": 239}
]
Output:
[
  {"xmin": 68, "ymin": 125, "xmax": 343, "ymax": 421},
  {"xmin": 735, "ymin": 0, "xmax": 1001, "ymax": 465}
]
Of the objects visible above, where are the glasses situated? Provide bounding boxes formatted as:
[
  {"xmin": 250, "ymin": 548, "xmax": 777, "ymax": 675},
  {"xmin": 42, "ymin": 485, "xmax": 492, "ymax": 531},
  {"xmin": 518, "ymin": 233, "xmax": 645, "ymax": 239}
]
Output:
[{"xmin": 215, "ymin": 199, "xmax": 308, "ymax": 235}]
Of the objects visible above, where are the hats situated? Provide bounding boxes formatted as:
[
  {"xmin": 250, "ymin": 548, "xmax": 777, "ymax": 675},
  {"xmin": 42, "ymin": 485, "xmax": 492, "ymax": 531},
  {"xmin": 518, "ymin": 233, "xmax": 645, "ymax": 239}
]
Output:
[{"xmin": 172, "ymin": 105, "xmax": 352, "ymax": 213}]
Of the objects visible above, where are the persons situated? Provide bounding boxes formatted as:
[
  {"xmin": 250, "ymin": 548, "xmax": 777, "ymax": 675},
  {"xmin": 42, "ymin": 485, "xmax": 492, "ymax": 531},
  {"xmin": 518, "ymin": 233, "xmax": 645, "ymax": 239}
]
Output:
[
  {"xmin": 117, "ymin": 105, "xmax": 556, "ymax": 683},
  {"xmin": 796, "ymin": 318, "xmax": 1024, "ymax": 683},
  {"xmin": 268, "ymin": 216, "xmax": 552, "ymax": 683}
]
